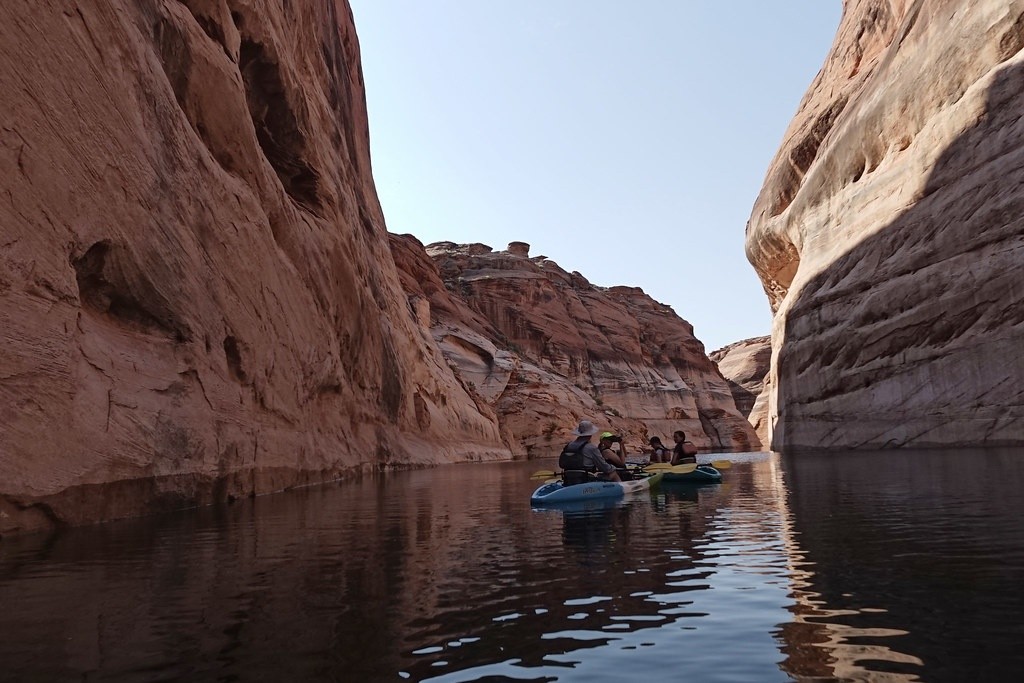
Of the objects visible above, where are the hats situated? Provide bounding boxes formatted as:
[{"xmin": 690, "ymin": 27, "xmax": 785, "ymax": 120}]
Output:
[
  {"xmin": 648, "ymin": 437, "xmax": 660, "ymax": 446},
  {"xmin": 600, "ymin": 431, "xmax": 613, "ymax": 441},
  {"xmin": 571, "ymin": 421, "xmax": 599, "ymax": 436}
]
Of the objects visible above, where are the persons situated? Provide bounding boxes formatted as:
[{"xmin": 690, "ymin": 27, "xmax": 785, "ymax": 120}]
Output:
[
  {"xmin": 558, "ymin": 421, "xmax": 628, "ymax": 486},
  {"xmin": 646, "ymin": 430, "xmax": 697, "ymax": 464}
]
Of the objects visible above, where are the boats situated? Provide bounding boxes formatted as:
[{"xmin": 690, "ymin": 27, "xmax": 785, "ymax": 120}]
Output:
[
  {"xmin": 530, "ymin": 468, "xmax": 664, "ymax": 506},
  {"xmin": 658, "ymin": 466, "xmax": 723, "ymax": 483}
]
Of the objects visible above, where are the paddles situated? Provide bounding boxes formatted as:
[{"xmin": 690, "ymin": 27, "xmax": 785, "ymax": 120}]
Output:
[{"xmin": 529, "ymin": 459, "xmax": 731, "ymax": 481}]
